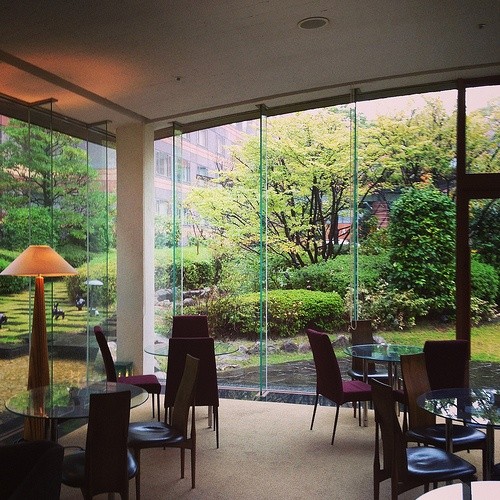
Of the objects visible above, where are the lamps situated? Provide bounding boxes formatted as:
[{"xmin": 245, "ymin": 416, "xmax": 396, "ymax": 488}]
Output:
[{"xmin": 0, "ymin": 245, "xmax": 80, "ymax": 445}]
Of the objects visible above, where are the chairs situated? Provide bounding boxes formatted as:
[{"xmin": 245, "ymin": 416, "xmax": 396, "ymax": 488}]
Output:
[
  {"xmin": 165, "ymin": 337, "xmax": 220, "ymax": 449},
  {"xmin": 93, "ymin": 326, "xmax": 161, "ymax": 423},
  {"xmin": 351, "ymin": 319, "xmax": 400, "ymax": 418},
  {"xmin": 60, "ymin": 391, "xmax": 140, "ymax": 500},
  {"xmin": 306, "ymin": 329, "xmax": 373, "ymax": 445},
  {"xmin": 108, "ymin": 354, "xmax": 199, "ymax": 500},
  {"xmin": 421, "ymin": 340, "xmax": 473, "ymax": 455},
  {"xmin": 371, "ymin": 377, "xmax": 477, "ymax": 500},
  {"xmin": 0, "ymin": 441, "xmax": 64, "ymax": 500},
  {"xmin": 398, "ymin": 354, "xmax": 489, "ymax": 482},
  {"xmin": 169, "ymin": 316, "xmax": 212, "ymax": 431}
]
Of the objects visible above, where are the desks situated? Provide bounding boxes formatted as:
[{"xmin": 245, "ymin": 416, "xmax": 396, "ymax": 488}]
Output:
[
  {"xmin": 415, "ymin": 386, "xmax": 500, "ymax": 481},
  {"xmin": 4, "ymin": 381, "xmax": 149, "ymax": 455},
  {"xmin": 347, "ymin": 344, "xmax": 426, "ymax": 421}
]
